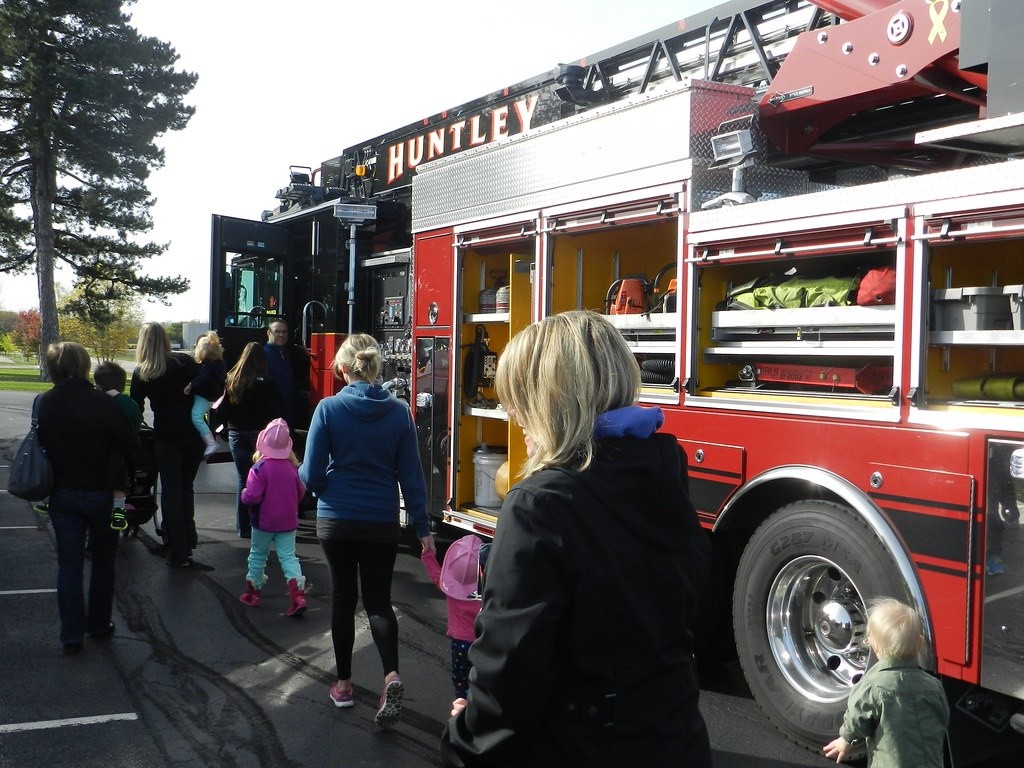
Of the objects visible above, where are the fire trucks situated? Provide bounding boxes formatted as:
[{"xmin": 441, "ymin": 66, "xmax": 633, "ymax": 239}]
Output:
[{"xmin": 206, "ymin": 0, "xmax": 1024, "ymax": 768}]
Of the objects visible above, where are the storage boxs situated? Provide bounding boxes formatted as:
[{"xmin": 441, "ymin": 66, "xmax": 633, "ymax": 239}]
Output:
[
  {"xmin": 1003, "ymin": 284, "xmax": 1024, "ymax": 330},
  {"xmin": 933, "ymin": 288, "xmax": 1013, "ymax": 330}
]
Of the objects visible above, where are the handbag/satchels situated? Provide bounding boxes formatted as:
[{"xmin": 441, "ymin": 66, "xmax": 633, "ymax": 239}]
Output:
[{"xmin": 7, "ymin": 392, "xmax": 54, "ymax": 502}]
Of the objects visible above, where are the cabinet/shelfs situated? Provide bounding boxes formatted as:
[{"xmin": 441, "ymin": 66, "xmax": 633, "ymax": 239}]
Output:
[{"xmin": 462, "ymin": 313, "xmax": 512, "ymax": 422}]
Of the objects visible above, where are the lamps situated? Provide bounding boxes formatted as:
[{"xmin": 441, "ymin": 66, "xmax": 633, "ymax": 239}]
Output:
[{"xmin": 700, "ymin": 110, "xmax": 758, "ymax": 208}]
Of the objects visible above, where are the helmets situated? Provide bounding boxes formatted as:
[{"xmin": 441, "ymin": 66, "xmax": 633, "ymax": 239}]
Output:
[
  {"xmin": 439, "ymin": 535, "xmax": 484, "ymax": 601},
  {"xmin": 256, "ymin": 418, "xmax": 293, "ymax": 459}
]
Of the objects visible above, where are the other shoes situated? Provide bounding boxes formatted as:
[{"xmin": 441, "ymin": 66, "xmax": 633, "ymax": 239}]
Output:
[
  {"xmin": 62, "ymin": 641, "xmax": 84, "ymax": 654},
  {"xmin": 451, "ymin": 697, "xmax": 467, "ymax": 716},
  {"xmin": 90, "ymin": 621, "xmax": 115, "ymax": 638},
  {"xmin": 175, "ymin": 548, "xmax": 194, "ymax": 567}
]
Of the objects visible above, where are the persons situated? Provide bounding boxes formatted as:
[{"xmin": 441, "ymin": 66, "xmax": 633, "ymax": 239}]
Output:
[
  {"xmin": 31, "ymin": 341, "xmax": 143, "ymax": 655},
  {"xmin": 421, "ymin": 535, "xmax": 485, "ymax": 716},
  {"xmin": 297, "ymin": 333, "xmax": 437, "ymax": 729},
  {"xmin": 240, "ymin": 417, "xmax": 308, "ymax": 616},
  {"xmin": 440, "ymin": 309, "xmax": 713, "ymax": 768},
  {"xmin": 226, "ymin": 342, "xmax": 294, "ymax": 539},
  {"xmin": 822, "ymin": 596, "xmax": 949, "ymax": 768},
  {"xmin": 261, "ymin": 318, "xmax": 306, "ymax": 519},
  {"xmin": 129, "ymin": 325, "xmax": 227, "ymax": 568}
]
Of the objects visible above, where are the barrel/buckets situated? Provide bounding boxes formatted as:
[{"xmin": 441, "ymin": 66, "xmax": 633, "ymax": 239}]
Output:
[{"xmin": 472, "ymin": 445, "xmax": 508, "ymax": 508}]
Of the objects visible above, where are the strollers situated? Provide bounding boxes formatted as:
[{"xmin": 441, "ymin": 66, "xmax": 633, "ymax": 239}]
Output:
[{"xmin": 81, "ymin": 418, "xmax": 173, "ymax": 553}]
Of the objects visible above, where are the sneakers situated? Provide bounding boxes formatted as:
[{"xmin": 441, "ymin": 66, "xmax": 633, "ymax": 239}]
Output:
[
  {"xmin": 34, "ymin": 496, "xmax": 51, "ymax": 513},
  {"xmin": 374, "ymin": 675, "xmax": 405, "ymax": 729},
  {"xmin": 110, "ymin": 506, "xmax": 129, "ymax": 530},
  {"xmin": 329, "ymin": 682, "xmax": 354, "ymax": 708}
]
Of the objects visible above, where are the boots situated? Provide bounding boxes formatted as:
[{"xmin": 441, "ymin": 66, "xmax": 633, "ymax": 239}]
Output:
[
  {"xmin": 240, "ymin": 574, "xmax": 269, "ymax": 606},
  {"xmin": 286, "ymin": 576, "xmax": 308, "ymax": 616},
  {"xmin": 202, "ymin": 431, "xmax": 220, "ymax": 457}
]
